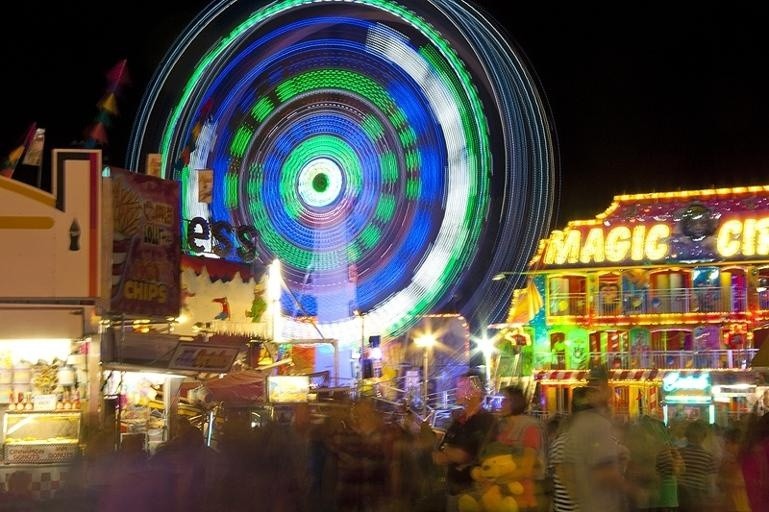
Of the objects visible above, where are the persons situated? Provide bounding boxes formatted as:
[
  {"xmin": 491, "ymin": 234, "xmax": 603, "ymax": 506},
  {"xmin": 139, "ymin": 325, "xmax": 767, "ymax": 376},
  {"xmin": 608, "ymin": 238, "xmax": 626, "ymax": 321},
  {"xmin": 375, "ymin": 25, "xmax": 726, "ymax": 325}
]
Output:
[
  {"xmin": 681, "ymin": 203, "xmax": 716, "ymax": 237},
  {"xmin": 80, "ymin": 373, "xmax": 769, "ymax": 512}
]
